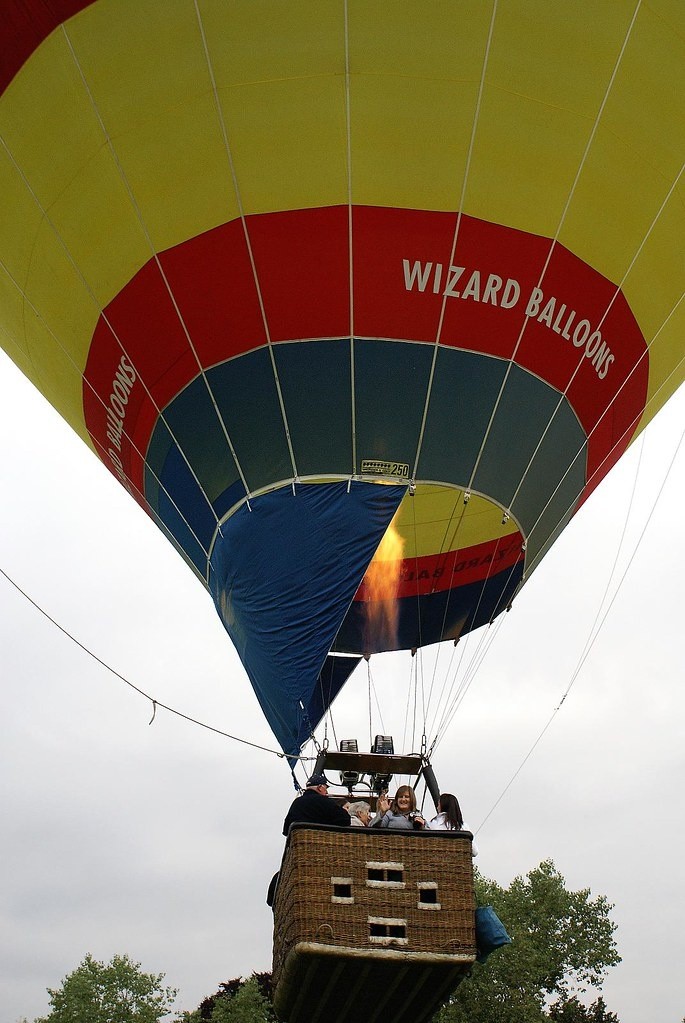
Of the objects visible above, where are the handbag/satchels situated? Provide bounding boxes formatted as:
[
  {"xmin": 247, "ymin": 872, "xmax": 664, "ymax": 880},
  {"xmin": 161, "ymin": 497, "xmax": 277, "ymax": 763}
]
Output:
[{"xmin": 474, "ymin": 906, "xmax": 512, "ymax": 964}]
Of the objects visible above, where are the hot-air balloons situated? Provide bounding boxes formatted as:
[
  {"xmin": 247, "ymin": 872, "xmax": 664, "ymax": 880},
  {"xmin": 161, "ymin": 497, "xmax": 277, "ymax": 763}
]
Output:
[{"xmin": 1, "ymin": 2, "xmax": 685, "ymax": 1022}]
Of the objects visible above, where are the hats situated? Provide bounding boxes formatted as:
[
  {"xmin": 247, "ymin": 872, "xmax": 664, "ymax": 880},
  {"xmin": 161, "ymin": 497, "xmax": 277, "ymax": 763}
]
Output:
[{"xmin": 307, "ymin": 774, "xmax": 330, "ymax": 789}]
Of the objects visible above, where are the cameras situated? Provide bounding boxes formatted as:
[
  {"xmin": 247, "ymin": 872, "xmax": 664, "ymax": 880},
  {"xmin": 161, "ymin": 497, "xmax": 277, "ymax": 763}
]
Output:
[{"xmin": 409, "ymin": 811, "xmax": 423, "ymax": 828}]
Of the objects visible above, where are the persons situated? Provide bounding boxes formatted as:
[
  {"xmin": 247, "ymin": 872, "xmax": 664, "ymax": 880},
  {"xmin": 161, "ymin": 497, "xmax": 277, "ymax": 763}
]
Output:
[
  {"xmin": 282, "ymin": 774, "xmax": 372, "ymax": 836},
  {"xmin": 368, "ymin": 785, "xmax": 428, "ymax": 830},
  {"xmin": 425, "ymin": 793, "xmax": 479, "ymax": 857}
]
